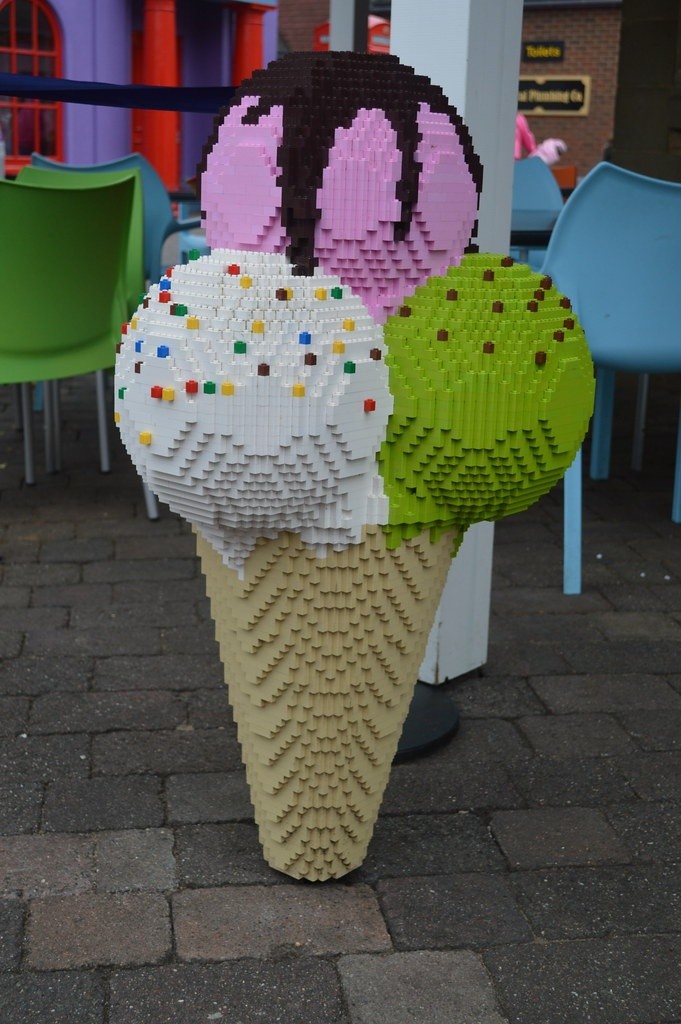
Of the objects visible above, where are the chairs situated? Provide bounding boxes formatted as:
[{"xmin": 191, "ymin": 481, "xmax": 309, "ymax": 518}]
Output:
[
  {"xmin": 176, "ymin": 202, "xmax": 211, "ymax": 265},
  {"xmin": 31, "ymin": 151, "xmax": 203, "ymax": 294},
  {"xmin": 509, "ymin": 155, "xmax": 563, "ymax": 268},
  {"xmin": 0, "ymin": 175, "xmax": 160, "ymax": 521},
  {"xmin": 534, "ymin": 160, "xmax": 680, "ymax": 597},
  {"xmin": 13, "ymin": 165, "xmax": 141, "ymax": 483}
]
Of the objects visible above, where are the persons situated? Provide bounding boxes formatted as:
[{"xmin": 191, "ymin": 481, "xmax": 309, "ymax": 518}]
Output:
[{"xmin": 514, "ymin": 110, "xmax": 537, "ymax": 159}]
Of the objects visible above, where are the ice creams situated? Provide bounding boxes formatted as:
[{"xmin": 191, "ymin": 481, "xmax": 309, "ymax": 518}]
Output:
[{"xmin": 111, "ymin": 50, "xmax": 598, "ymax": 884}]
